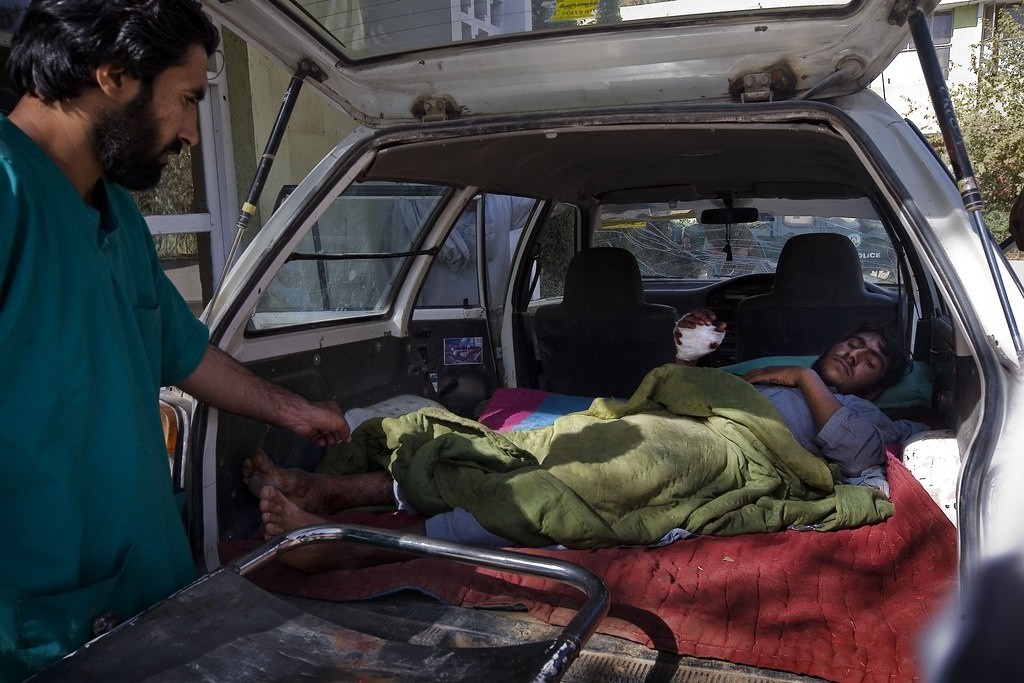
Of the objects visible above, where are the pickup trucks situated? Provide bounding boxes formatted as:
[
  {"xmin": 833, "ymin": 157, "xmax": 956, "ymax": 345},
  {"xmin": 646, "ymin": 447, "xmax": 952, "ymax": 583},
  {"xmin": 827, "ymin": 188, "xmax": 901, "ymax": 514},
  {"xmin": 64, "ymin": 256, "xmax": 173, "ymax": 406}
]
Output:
[{"xmin": 727, "ymin": 217, "xmax": 863, "ymax": 248}]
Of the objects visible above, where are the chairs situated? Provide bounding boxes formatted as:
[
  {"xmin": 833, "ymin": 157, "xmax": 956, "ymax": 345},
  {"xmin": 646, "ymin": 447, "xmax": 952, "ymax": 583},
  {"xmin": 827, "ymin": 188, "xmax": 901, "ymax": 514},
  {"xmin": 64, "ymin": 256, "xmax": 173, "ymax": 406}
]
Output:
[
  {"xmin": 732, "ymin": 231, "xmax": 904, "ymax": 366},
  {"xmin": 533, "ymin": 245, "xmax": 683, "ymax": 406}
]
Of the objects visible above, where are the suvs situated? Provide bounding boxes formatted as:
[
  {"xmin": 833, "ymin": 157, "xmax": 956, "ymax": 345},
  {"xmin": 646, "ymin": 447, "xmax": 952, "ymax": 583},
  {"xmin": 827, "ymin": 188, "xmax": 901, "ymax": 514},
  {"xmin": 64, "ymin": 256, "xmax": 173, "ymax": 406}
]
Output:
[{"xmin": 164, "ymin": 0, "xmax": 1024, "ymax": 682}]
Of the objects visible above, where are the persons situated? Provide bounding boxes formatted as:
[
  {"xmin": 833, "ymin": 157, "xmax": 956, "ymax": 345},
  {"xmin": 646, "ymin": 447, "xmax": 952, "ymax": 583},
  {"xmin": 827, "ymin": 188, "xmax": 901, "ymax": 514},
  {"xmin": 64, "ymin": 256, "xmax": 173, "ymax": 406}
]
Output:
[
  {"xmin": 386, "ymin": 193, "xmax": 536, "ymax": 307},
  {"xmin": 241, "ymin": 307, "xmax": 915, "ymax": 578},
  {"xmin": 1, "ymin": 0, "xmax": 353, "ymax": 683}
]
